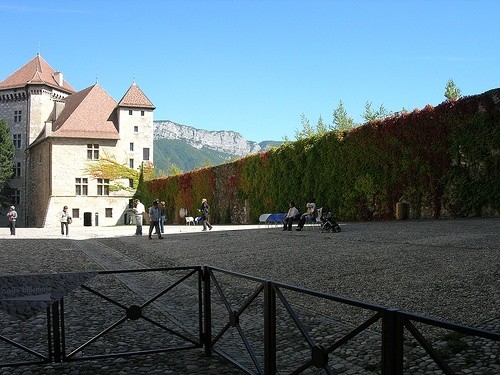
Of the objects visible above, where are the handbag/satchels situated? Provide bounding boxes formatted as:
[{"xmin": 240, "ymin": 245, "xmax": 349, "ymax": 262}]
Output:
[{"xmin": 67, "ymin": 217, "xmax": 72, "ymax": 223}]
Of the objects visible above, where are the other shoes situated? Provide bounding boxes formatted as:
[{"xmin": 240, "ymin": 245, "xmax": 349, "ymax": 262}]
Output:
[
  {"xmin": 296, "ymin": 228, "xmax": 301, "ymax": 231},
  {"xmin": 282, "ymin": 229, "xmax": 287, "ymax": 230},
  {"xmin": 159, "ymin": 236, "xmax": 163, "ymax": 239},
  {"xmin": 149, "ymin": 237, "xmax": 152, "ymax": 239}
]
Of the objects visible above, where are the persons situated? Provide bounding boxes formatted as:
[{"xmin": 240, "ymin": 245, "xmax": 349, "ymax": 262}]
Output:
[
  {"xmin": 153, "ymin": 201, "xmax": 166, "ymax": 235},
  {"xmin": 282, "ymin": 203, "xmax": 300, "ymax": 231},
  {"xmin": 296, "ymin": 203, "xmax": 317, "ymax": 231},
  {"xmin": 7, "ymin": 206, "xmax": 18, "ymax": 235},
  {"xmin": 60, "ymin": 206, "xmax": 71, "ymax": 235},
  {"xmin": 132, "ymin": 199, "xmax": 146, "ymax": 236},
  {"xmin": 148, "ymin": 201, "xmax": 164, "ymax": 240},
  {"xmin": 201, "ymin": 198, "xmax": 212, "ymax": 231}
]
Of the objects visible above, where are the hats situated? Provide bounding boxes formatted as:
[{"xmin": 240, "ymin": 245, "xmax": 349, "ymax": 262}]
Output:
[
  {"xmin": 11, "ymin": 206, "xmax": 15, "ymax": 209},
  {"xmin": 153, "ymin": 201, "xmax": 158, "ymax": 205}
]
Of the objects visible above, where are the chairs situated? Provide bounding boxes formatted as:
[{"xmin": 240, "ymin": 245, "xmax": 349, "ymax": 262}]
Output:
[
  {"xmin": 259, "ymin": 214, "xmax": 314, "ymax": 232},
  {"xmin": 185, "ymin": 217, "xmax": 195, "ymax": 226}
]
